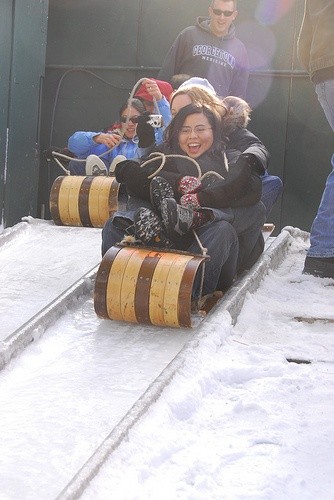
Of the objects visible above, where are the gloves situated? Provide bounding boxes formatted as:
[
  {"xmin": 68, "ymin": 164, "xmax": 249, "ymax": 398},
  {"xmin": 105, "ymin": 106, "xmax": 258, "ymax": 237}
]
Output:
[
  {"xmin": 177, "ymin": 175, "xmax": 202, "ymax": 195},
  {"xmin": 180, "ymin": 193, "xmax": 201, "ymax": 207},
  {"xmin": 136, "ymin": 111, "xmax": 154, "ymax": 147},
  {"xmin": 121, "ymin": 163, "xmax": 148, "ymax": 196},
  {"xmin": 224, "ymin": 154, "xmax": 252, "ymax": 197}
]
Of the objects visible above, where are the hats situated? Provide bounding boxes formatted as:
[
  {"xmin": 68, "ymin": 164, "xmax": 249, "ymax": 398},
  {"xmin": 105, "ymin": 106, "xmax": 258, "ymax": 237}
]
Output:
[
  {"xmin": 134, "ymin": 78, "xmax": 173, "ymax": 105},
  {"xmin": 170, "ymin": 77, "xmax": 216, "ymax": 108}
]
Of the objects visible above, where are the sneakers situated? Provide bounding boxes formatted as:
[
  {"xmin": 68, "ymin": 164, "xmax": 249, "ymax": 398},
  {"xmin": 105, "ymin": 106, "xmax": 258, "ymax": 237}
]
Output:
[
  {"xmin": 149, "ymin": 176, "xmax": 194, "ymax": 229},
  {"xmin": 161, "ymin": 199, "xmax": 193, "ymax": 250}
]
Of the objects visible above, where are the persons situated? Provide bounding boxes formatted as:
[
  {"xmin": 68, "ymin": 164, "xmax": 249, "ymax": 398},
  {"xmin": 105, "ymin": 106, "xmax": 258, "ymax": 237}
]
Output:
[
  {"xmin": 297, "ymin": 0, "xmax": 334, "ymax": 279},
  {"xmin": 100, "ymin": 74, "xmax": 283, "ymax": 297},
  {"xmin": 65, "ymin": 78, "xmax": 173, "ymax": 175},
  {"xmin": 158, "ymin": 0, "xmax": 250, "ymax": 102}
]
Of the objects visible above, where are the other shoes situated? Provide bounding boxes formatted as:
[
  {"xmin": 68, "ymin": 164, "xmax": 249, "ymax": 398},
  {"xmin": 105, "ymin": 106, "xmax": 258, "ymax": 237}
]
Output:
[
  {"xmin": 133, "ymin": 207, "xmax": 175, "ymax": 248},
  {"xmin": 302, "ymin": 257, "xmax": 334, "ymax": 278},
  {"xmin": 85, "ymin": 153, "xmax": 127, "ymax": 191},
  {"xmin": 199, "ymin": 292, "xmax": 218, "ymax": 314}
]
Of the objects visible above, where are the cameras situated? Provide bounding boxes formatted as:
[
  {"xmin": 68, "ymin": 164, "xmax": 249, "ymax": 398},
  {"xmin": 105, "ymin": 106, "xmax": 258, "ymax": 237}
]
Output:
[{"xmin": 147, "ymin": 114, "xmax": 162, "ymax": 128}]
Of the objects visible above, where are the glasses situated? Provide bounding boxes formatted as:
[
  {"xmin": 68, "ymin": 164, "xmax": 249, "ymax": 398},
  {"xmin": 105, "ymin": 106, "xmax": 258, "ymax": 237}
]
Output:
[
  {"xmin": 120, "ymin": 117, "xmax": 139, "ymax": 123},
  {"xmin": 210, "ymin": 7, "xmax": 236, "ymax": 17}
]
